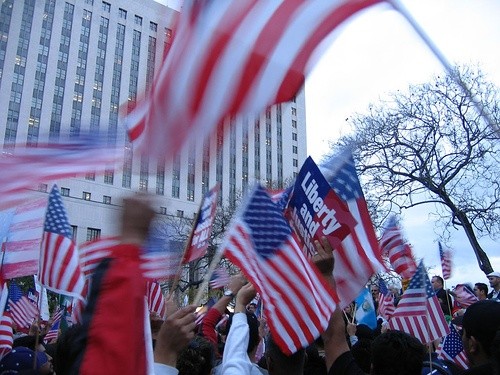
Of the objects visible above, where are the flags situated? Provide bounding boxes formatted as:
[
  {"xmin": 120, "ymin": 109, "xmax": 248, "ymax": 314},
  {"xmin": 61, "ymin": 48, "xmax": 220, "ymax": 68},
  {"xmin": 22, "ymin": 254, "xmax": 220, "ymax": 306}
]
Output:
[{"xmin": 0, "ymin": 0, "xmax": 481, "ymax": 371}]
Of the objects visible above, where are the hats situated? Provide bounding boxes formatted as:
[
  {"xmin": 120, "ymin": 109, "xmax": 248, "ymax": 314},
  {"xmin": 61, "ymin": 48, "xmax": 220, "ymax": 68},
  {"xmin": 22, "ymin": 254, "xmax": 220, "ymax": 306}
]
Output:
[
  {"xmin": 487, "ymin": 271, "xmax": 500, "ymax": 277},
  {"xmin": 453, "ymin": 298, "xmax": 500, "ymax": 333},
  {"xmin": 0, "ymin": 346, "xmax": 47, "ymax": 370}
]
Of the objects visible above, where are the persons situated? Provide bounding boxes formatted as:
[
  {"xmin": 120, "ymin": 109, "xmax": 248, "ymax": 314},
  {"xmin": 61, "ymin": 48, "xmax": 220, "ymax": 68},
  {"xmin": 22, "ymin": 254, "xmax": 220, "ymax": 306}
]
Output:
[{"xmin": 0, "ymin": 186, "xmax": 500, "ymax": 375}]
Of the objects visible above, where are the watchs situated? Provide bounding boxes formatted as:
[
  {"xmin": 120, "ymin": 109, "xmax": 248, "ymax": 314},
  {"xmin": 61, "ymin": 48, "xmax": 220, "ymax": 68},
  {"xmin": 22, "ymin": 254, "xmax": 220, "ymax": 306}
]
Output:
[{"xmin": 224, "ymin": 289, "xmax": 235, "ymax": 299}]
